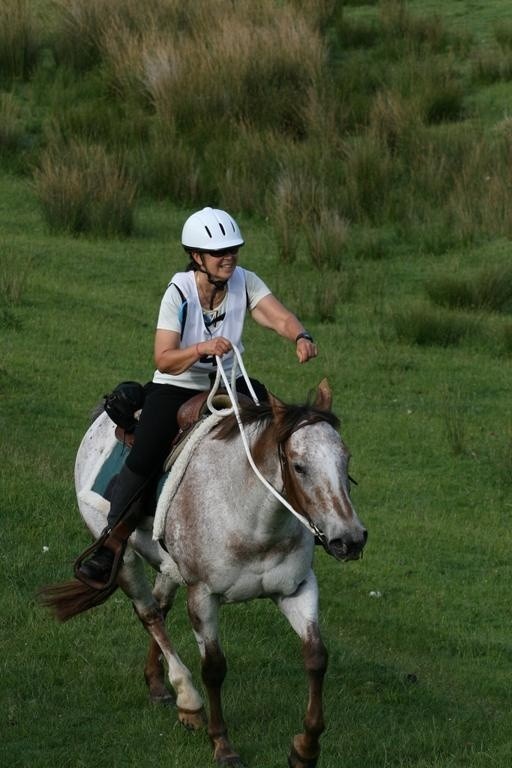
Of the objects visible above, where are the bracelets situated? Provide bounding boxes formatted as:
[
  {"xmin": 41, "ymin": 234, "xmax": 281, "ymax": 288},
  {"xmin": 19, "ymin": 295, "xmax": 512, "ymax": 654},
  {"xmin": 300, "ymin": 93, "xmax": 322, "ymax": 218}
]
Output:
[{"xmin": 196, "ymin": 342, "xmax": 205, "ymax": 357}]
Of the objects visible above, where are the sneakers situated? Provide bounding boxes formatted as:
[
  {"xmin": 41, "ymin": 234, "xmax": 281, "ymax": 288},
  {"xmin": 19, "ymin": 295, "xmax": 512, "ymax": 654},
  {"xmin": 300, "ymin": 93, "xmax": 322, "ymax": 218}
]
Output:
[{"xmin": 76, "ymin": 537, "xmax": 125, "ymax": 590}]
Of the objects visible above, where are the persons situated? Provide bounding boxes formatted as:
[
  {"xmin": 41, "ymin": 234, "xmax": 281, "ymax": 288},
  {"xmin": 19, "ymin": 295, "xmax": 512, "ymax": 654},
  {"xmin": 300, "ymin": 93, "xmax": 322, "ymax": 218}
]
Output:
[{"xmin": 77, "ymin": 204, "xmax": 320, "ymax": 584}]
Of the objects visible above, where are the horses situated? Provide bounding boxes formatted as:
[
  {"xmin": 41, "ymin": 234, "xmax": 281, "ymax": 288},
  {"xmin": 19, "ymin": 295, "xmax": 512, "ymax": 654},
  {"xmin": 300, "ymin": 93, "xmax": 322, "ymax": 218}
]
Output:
[{"xmin": 42, "ymin": 377, "xmax": 369, "ymax": 768}]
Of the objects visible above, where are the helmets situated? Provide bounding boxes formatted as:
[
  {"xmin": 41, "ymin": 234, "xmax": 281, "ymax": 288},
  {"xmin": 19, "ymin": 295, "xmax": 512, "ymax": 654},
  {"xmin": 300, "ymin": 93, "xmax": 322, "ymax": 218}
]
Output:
[{"xmin": 180, "ymin": 207, "xmax": 244, "ymax": 252}]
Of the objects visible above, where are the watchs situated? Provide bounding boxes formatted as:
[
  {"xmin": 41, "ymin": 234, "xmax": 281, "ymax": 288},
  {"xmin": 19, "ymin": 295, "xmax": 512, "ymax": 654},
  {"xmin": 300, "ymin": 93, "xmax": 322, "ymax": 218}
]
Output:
[{"xmin": 295, "ymin": 332, "xmax": 315, "ymax": 344}]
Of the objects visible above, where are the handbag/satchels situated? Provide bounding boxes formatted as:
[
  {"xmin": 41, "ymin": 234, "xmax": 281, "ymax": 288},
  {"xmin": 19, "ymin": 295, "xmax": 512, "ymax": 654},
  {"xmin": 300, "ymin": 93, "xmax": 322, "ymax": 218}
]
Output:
[{"xmin": 103, "ymin": 378, "xmax": 151, "ymax": 433}]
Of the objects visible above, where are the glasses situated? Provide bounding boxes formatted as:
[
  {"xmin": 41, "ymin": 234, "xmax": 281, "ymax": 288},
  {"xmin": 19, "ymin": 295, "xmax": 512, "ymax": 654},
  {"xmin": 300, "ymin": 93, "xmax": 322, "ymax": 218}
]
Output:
[{"xmin": 200, "ymin": 246, "xmax": 239, "ymax": 257}]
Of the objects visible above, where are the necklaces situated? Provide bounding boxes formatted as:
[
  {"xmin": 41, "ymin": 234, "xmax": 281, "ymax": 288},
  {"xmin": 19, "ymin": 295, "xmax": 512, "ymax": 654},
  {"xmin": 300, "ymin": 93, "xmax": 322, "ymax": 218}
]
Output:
[{"xmin": 197, "ymin": 271, "xmax": 227, "ymax": 305}]
